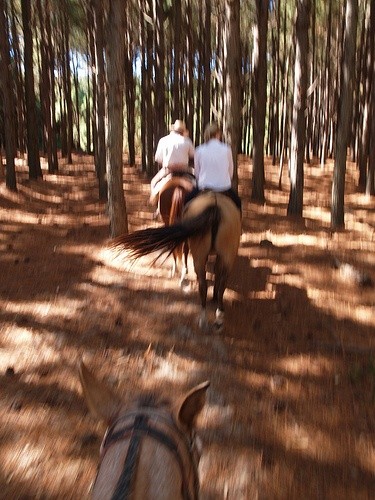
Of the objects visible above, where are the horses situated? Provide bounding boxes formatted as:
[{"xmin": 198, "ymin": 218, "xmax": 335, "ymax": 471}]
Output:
[
  {"xmin": 103, "ymin": 191, "xmax": 241, "ymax": 333},
  {"xmin": 157, "ymin": 177, "xmax": 190, "ymax": 287},
  {"xmin": 75, "ymin": 360, "xmax": 211, "ymax": 499}
]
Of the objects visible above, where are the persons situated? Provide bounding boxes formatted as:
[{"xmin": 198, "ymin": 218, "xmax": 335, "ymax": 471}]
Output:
[
  {"xmin": 151, "ymin": 119, "xmax": 195, "ymax": 192},
  {"xmin": 183, "ymin": 123, "xmax": 243, "ymax": 219}
]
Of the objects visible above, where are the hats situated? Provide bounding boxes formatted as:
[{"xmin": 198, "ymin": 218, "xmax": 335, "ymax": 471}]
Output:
[{"xmin": 170, "ymin": 119, "xmax": 186, "ymax": 133}]
